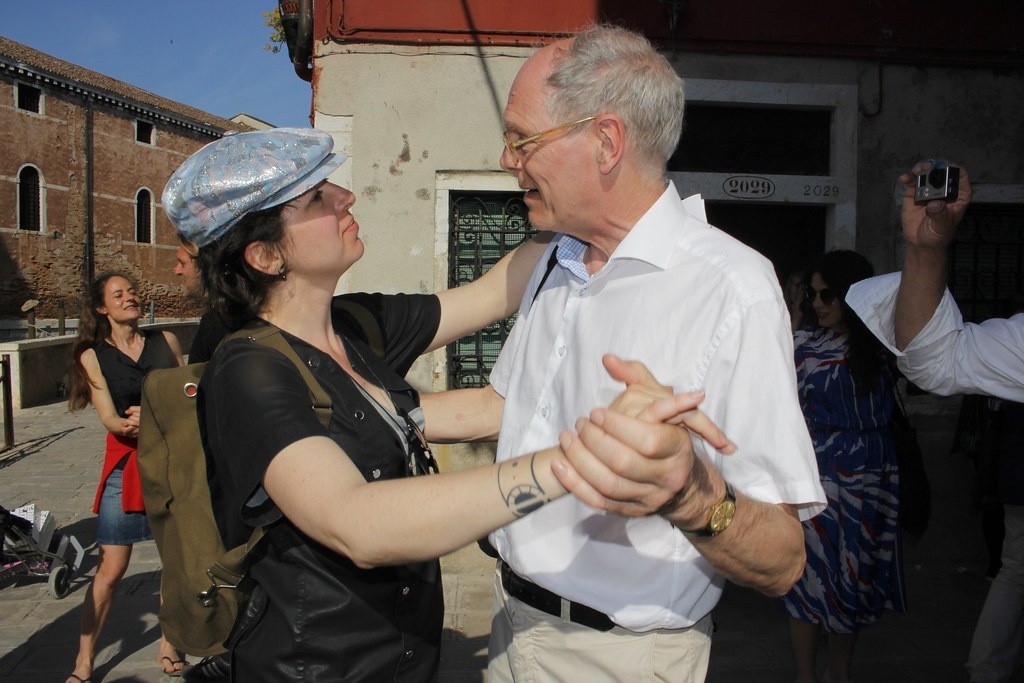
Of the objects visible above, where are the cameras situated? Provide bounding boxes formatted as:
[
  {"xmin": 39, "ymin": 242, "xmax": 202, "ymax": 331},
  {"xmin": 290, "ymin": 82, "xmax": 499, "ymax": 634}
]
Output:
[{"xmin": 914, "ymin": 165, "xmax": 960, "ymax": 205}]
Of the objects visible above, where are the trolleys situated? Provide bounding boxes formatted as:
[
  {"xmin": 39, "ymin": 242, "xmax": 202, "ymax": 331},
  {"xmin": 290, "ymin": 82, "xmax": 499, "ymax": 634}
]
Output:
[{"xmin": 0, "ymin": 502, "xmax": 86, "ymax": 601}]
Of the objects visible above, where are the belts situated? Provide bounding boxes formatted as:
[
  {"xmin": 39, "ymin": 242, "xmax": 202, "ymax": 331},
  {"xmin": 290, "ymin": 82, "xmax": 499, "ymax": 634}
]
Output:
[{"xmin": 498, "ymin": 559, "xmax": 617, "ymax": 633}]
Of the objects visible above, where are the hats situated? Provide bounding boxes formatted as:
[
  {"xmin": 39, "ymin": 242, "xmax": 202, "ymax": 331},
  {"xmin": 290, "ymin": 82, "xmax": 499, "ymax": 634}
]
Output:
[{"xmin": 160, "ymin": 126, "xmax": 350, "ymax": 249}]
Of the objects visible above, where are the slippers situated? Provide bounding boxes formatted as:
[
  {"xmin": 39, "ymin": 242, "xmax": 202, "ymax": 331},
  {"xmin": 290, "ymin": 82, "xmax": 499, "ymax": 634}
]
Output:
[
  {"xmin": 161, "ymin": 657, "xmax": 190, "ymax": 676},
  {"xmin": 63, "ymin": 674, "xmax": 95, "ymax": 683}
]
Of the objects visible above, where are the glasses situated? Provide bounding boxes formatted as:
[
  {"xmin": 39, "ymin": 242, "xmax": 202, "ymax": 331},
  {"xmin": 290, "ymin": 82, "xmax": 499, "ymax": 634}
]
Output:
[
  {"xmin": 397, "ymin": 407, "xmax": 439, "ymax": 476},
  {"xmin": 803, "ymin": 282, "xmax": 841, "ymax": 306},
  {"xmin": 502, "ymin": 115, "xmax": 597, "ymax": 163}
]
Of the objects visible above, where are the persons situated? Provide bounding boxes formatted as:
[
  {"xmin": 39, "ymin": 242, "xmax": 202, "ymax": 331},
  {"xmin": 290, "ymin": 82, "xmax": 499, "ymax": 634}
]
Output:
[
  {"xmin": 162, "ymin": 126, "xmax": 733, "ymax": 683},
  {"xmin": 174, "ymin": 233, "xmax": 235, "ymax": 366},
  {"xmin": 416, "ymin": 26, "xmax": 827, "ymax": 683},
  {"xmin": 778, "ymin": 250, "xmax": 904, "ymax": 683},
  {"xmin": 846, "ymin": 158, "xmax": 1024, "ymax": 683},
  {"xmin": 67, "ymin": 273, "xmax": 187, "ymax": 683}
]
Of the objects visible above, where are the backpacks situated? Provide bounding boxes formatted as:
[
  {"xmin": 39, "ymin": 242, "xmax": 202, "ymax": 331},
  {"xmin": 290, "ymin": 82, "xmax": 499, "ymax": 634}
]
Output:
[{"xmin": 137, "ymin": 294, "xmax": 390, "ymax": 659}]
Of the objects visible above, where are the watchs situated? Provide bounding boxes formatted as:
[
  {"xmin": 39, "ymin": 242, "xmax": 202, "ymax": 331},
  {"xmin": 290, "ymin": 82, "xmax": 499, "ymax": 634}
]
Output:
[{"xmin": 682, "ymin": 481, "xmax": 736, "ymax": 537}]
